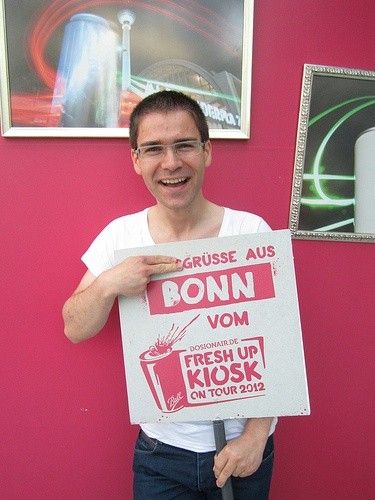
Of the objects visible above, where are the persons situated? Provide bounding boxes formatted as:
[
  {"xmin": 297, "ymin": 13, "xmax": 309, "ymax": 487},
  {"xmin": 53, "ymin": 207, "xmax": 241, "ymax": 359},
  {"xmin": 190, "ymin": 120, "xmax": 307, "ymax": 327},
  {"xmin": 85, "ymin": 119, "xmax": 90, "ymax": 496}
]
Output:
[{"xmin": 62, "ymin": 91, "xmax": 279, "ymax": 500}]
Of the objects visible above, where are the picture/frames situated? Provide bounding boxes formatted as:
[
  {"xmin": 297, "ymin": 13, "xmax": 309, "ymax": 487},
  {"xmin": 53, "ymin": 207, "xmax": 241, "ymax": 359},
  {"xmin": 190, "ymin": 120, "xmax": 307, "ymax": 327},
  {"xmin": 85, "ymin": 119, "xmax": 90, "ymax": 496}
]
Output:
[
  {"xmin": 287, "ymin": 63, "xmax": 375, "ymax": 243},
  {"xmin": 0, "ymin": 0, "xmax": 254, "ymax": 139}
]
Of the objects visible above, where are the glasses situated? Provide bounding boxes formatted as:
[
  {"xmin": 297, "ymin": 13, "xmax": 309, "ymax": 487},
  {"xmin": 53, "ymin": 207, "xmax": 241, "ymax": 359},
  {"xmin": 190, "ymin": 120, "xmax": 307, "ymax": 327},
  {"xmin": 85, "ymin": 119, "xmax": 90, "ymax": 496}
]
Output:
[{"xmin": 135, "ymin": 140, "xmax": 205, "ymax": 161}]
